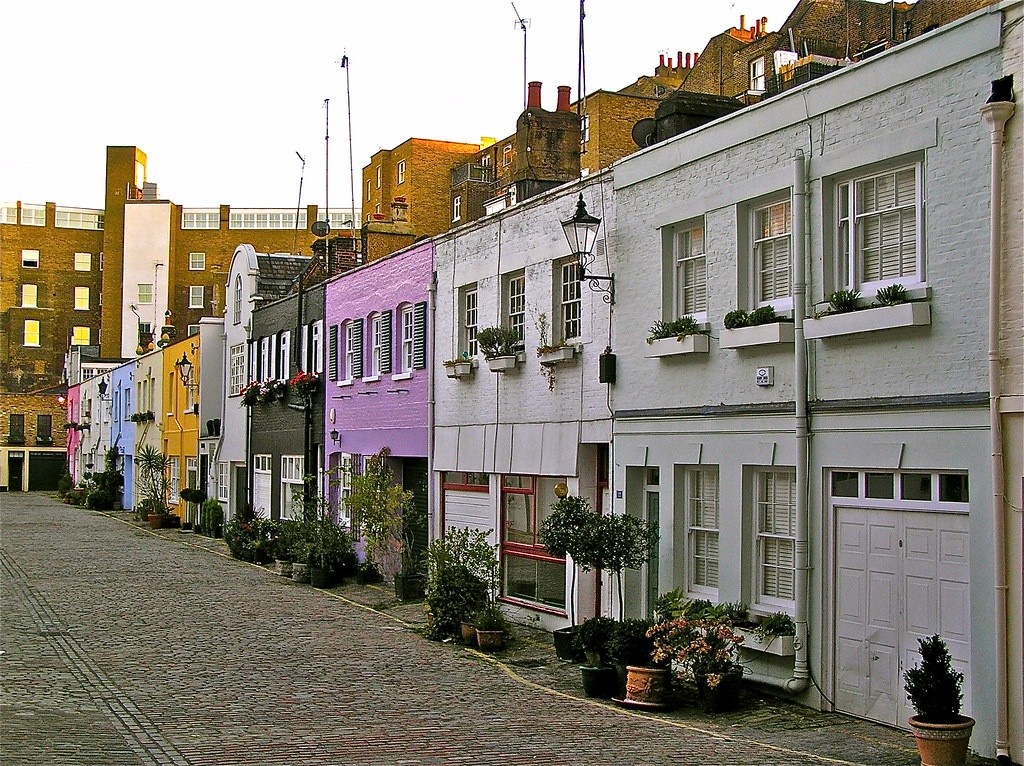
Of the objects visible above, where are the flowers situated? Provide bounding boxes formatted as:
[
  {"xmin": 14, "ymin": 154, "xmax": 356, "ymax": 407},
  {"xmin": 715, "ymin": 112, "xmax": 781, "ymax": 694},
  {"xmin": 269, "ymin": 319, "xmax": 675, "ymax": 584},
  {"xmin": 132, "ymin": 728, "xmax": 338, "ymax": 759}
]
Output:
[
  {"xmin": 291, "ymin": 370, "xmax": 319, "ymax": 396},
  {"xmin": 257, "ymin": 377, "xmax": 290, "ymax": 402},
  {"xmin": 239, "ymin": 381, "xmax": 260, "ymax": 405}
]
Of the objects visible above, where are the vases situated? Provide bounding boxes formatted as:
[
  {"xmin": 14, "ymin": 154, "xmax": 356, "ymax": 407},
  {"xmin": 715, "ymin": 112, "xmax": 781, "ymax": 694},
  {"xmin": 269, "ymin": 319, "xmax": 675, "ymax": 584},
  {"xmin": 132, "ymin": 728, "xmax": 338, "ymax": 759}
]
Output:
[{"xmin": 274, "ymin": 390, "xmax": 285, "ymax": 400}]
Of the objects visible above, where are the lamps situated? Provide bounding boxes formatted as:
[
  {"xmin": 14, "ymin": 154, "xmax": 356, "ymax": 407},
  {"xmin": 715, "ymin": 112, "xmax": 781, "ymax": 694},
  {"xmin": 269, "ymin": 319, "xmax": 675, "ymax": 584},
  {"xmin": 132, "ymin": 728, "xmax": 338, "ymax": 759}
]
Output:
[
  {"xmin": 98, "ymin": 375, "xmax": 115, "ymax": 402},
  {"xmin": 178, "ymin": 351, "xmax": 200, "ymax": 387},
  {"xmin": 57, "ymin": 393, "xmax": 68, "ymax": 411},
  {"xmin": 559, "ymin": 192, "xmax": 620, "ymax": 307}
]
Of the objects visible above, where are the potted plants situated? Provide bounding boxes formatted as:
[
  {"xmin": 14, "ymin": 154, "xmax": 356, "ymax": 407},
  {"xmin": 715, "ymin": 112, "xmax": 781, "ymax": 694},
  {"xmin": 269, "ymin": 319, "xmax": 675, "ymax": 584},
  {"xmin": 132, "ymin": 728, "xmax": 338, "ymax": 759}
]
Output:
[
  {"xmin": 291, "ymin": 536, "xmax": 311, "ymax": 584},
  {"xmin": 576, "ymin": 616, "xmax": 624, "ymax": 701},
  {"xmin": 200, "ymin": 501, "xmax": 207, "ymax": 535},
  {"xmin": 273, "ymin": 525, "xmax": 291, "ymax": 578},
  {"xmin": 656, "ymin": 590, "xmax": 797, "ymax": 658},
  {"xmin": 310, "ymin": 523, "xmax": 338, "ymax": 589},
  {"xmin": 139, "ymin": 445, "xmax": 158, "ymax": 523},
  {"xmin": 646, "ymin": 603, "xmax": 748, "ymax": 711},
  {"xmin": 180, "ymin": 487, "xmax": 194, "ymax": 530},
  {"xmin": 190, "ymin": 489, "xmax": 205, "ymax": 534},
  {"xmin": 477, "ymin": 325, "xmax": 518, "ymax": 368},
  {"xmin": 146, "ymin": 446, "xmax": 167, "ymax": 529},
  {"xmin": 131, "ymin": 409, "xmax": 156, "ymax": 426},
  {"xmin": 718, "ymin": 303, "xmax": 794, "ymax": 350},
  {"xmin": 458, "ymin": 585, "xmax": 484, "ymax": 646},
  {"xmin": 206, "ymin": 497, "xmax": 217, "ymax": 537},
  {"xmin": 534, "ymin": 312, "xmax": 575, "ymax": 393},
  {"xmin": 59, "ymin": 469, "xmax": 125, "ymax": 511},
  {"xmin": 644, "ymin": 314, "xmax": 710, "ymax": 358},
  {"xmin": 902, "ymin": 631, "xmax": 976, "ymax": 766},
  {"xmin": 474, "ymin": 562, "xmax": 505, "ymax": 649},
  {"xmin": 802, "ymin": 284, "xmax": 933, "ymax": 340},
  {"xmin": 614, "ymin": 619, "xmax": 672, "ymax": 713},
  {"xmin": 62, "ymin": 422, "xmax": 91, "ymax": 434},
  {"xmin": 442, "ymin": 356, "xmax": 472, "ymax": 379},
  {"xmin": 539, "ymin": 493, "xmax": 659, "ymax": 667},
  {"xmin": 393, "ymin": 530, "xmax": 425, "ymax": 601},
  {"xmin": 209, "ymin": 505, "xmax": 224, "ymax": 538}
]
